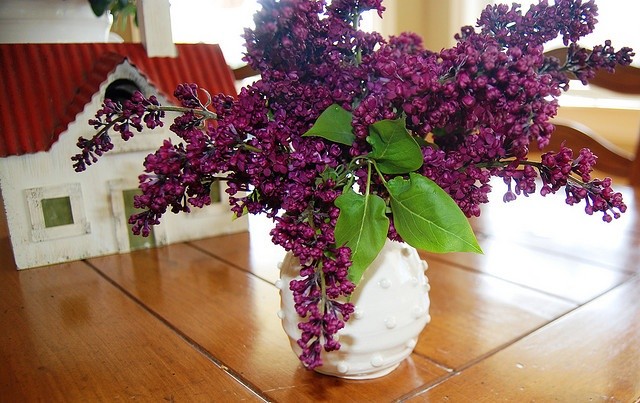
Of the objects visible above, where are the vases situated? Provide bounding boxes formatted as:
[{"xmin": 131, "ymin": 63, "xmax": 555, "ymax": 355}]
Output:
[{"xmin": 275, "ymin": 236, "xmax": 431, "ymax": 381}]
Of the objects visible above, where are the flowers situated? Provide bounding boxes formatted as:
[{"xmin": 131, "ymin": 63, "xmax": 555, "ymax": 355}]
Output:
[{"xmin": 70, "ymin": 0, "xmax": 636, "ymax": 373}]
